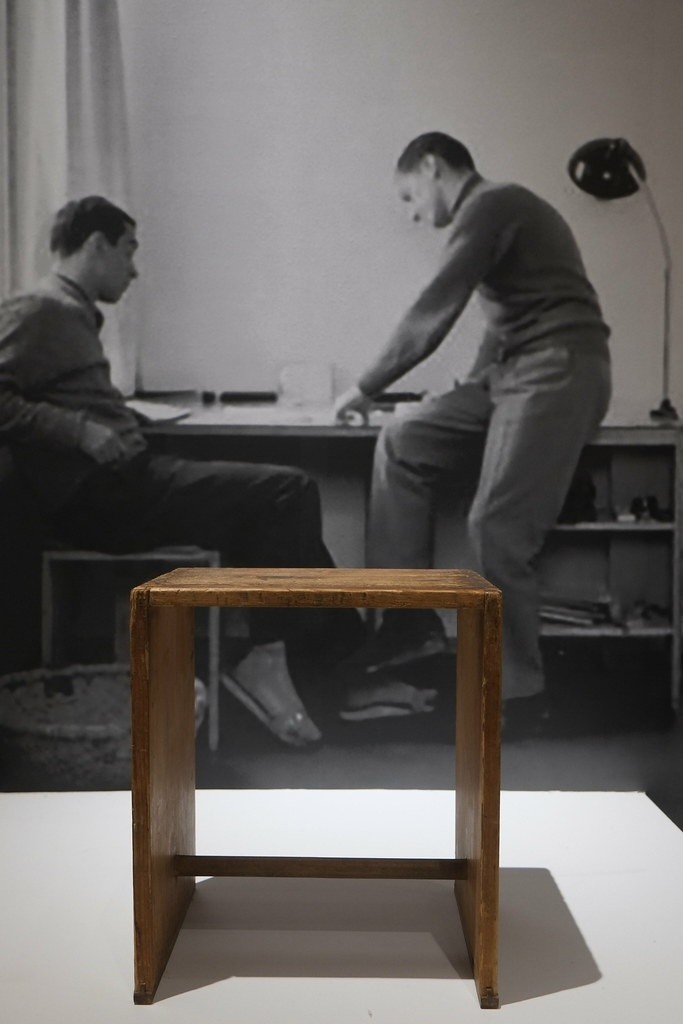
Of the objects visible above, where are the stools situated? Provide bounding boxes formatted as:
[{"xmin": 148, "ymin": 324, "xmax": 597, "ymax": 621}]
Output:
[{"xmin": 40, "ymin": 546, "xmax": 223, "ymax": 759}]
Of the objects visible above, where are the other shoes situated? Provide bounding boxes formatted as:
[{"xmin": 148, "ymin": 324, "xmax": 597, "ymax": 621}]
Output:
[
  {"xmin": 499, "ymin": 693, "xmax": 551, "ymax": 733},
  {"xmin": 339, "ymin": 676, "xmax": 438, "ymax": 718}
]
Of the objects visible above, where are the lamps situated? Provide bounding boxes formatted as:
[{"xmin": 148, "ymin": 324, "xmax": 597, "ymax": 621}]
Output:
[{"xmin": 567, "ymin": 136, "xmax": 681, "ymax": 422}]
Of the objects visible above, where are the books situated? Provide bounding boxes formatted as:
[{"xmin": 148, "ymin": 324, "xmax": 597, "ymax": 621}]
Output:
[
  {"xmin": 537, "ymin": 597, "xmax": 607, "ymax": 625},
  {"xmin": 124, "ymin": 399, "xmax": 190, "ymax": 423}
]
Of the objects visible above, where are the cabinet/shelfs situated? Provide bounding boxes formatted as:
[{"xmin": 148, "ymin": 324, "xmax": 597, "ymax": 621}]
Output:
[{"xmin": 538, "ymin": 425, "xmax": 683, "ymax": 711}]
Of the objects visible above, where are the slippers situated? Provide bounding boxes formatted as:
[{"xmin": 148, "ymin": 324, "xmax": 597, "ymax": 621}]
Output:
[{"xmin": 222, "ymin": 672, "xmax": 310, "ymax": 744}]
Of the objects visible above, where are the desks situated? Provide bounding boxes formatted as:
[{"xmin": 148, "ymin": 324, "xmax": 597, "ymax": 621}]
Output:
[
  {"xmin": 127, "ymin": 567, "xmax": 502, "ymax": 1010},
  {"xmin": 136, "ymin": 407, "xmax": 393, "ymax": 436},
  {"xmin": 0, "ymin": 788, "xmax": 683, "ymax": 1024}
]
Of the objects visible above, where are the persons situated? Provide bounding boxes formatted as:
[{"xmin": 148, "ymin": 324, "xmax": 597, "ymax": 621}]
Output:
[
  {"xmin": 333, "ymin": 131, "xmax": 611, "ymax": 732},
  {"xmin": 0, "ymin": 194, "xmax": 324, "ymax": 747}
]
